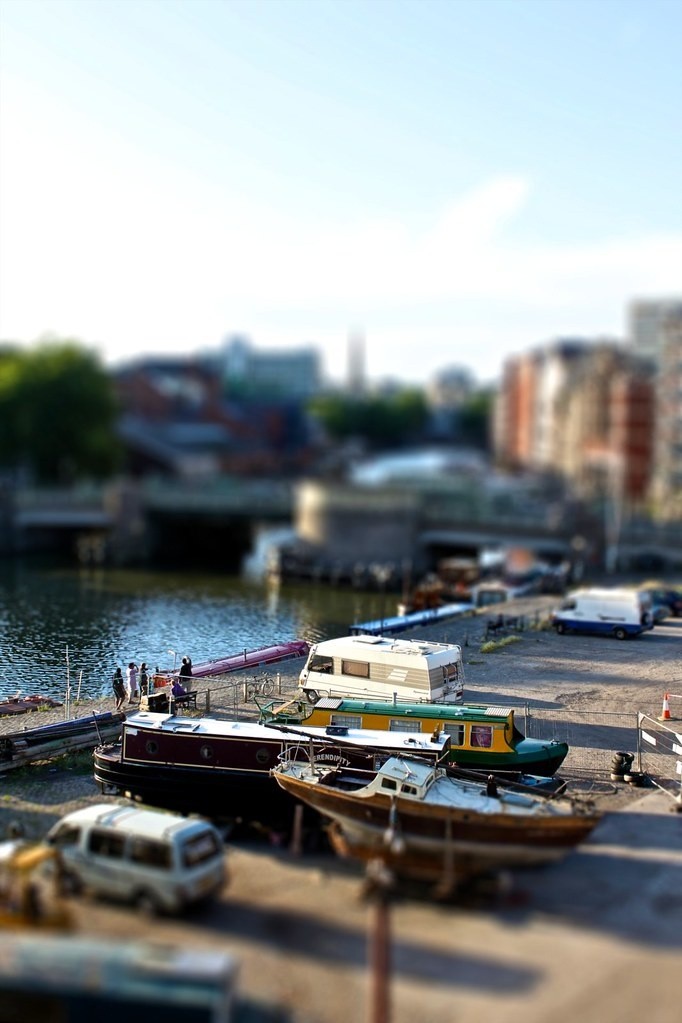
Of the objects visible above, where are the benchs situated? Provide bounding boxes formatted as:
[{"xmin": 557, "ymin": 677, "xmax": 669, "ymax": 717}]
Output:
[
  {"xmin": 169, "ymin": 691, "xmax": 198, "ymax": 709},
  {"xmin": 489, "ymin": 617, "xmax": 517, "ymax": 637}
]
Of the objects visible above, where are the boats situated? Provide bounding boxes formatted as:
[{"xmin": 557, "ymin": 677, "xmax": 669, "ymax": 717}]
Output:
[
  {"xmin": 0, "ymin": 693, "xmax": 65, "ymax": 719},
  {"xmin": 348, "ymin": 599, "xmax": 474, "ymax": 638},
  {"xmin": 89, "ymin": 689, "xmax": 573, "ymax": 830},
  {"xmin": 173, "ymin": 637, "xmax": 306, "ymax": 679},
  {"xmin": 262, "ymin": 718, "xmax": 600, "ymax": 887}
]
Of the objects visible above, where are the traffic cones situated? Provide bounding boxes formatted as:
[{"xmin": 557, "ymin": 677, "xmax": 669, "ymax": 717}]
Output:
[{"xmin": 657, "ymin": 692, "xmax": 676, "ymax": 722}]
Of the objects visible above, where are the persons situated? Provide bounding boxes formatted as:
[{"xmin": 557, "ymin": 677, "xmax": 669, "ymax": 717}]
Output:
[
  {"xmin": 448, "ymin": 761, "xmax": 452, "ymax": 766},
  {"xmin": 487, "ymin": 774, "xmax": 500, "ymax": 798},
  {"xmin": 141, "ymin": 686, "xmax": 148, "ymax": 696},
  {"xmin": 171, "ymin": 681, "xmax": 186, "ymax": 707},
  {"xmin": 126, "ymin": 663, "xmax": 138, "ymax": 703},
  {"xmin": 139, "ymin": 662, "xmax": 148, "ymax": 686},
  {"xmin": 112, "ymin": 668, "xmax": 126, "ymax": 711},
  {"xmin": 178, "ymin": 655, "xmax": 192, "ymax": 692},
  {"xmin": 451, "ymin": 761, "xmax": 458, "ymax": 767}
]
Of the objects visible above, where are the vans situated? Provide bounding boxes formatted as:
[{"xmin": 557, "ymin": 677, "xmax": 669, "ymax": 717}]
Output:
[
  {"xmin": 41, "ymin": 805, "xmax": 233, "ymax": 922},
  {"xmin": 546, "ymin": 589, "xmax": 656, "ymax": 640},
  {"xmin": 299, "ymin": 632, "xmax": 464, "ymax": 705}
]
